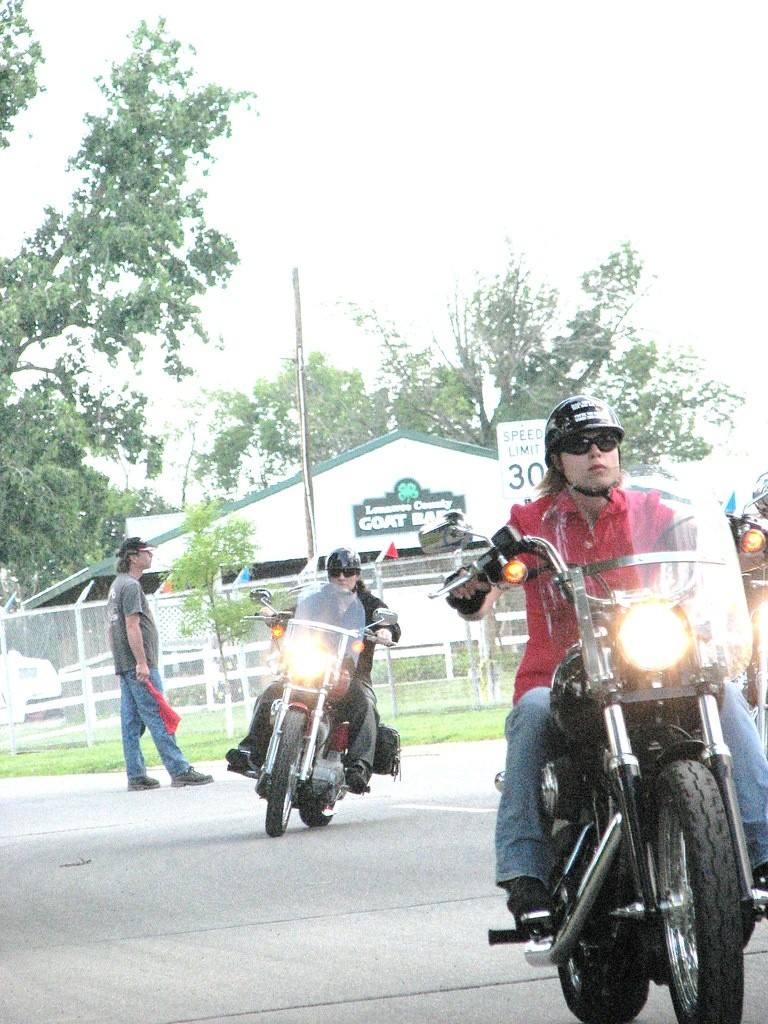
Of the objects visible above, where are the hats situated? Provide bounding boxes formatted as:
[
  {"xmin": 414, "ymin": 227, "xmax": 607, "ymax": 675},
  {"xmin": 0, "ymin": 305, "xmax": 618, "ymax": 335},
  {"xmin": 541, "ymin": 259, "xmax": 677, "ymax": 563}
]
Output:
[{"xmin": 118, "ymin": 538, "xmax": 157, "ymax": 557}]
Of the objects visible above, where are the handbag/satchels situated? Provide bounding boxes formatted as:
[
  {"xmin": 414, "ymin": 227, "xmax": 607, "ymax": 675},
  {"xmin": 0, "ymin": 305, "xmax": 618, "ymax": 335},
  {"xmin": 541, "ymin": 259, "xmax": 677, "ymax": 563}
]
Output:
[{"xmin": 371, "ymin": 724, "xmax": 400, "ymax": 775}]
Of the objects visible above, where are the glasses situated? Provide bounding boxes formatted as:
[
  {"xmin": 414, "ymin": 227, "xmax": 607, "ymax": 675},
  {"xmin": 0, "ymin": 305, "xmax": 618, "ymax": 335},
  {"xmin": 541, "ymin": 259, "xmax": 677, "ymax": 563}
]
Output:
[
  {"xmin": 558, "ymin": 431, "xmax": 619, "ymax": 455},
  {"xmin": 328, "ymin": 569, "xmax": 359, "ymax": 577}
]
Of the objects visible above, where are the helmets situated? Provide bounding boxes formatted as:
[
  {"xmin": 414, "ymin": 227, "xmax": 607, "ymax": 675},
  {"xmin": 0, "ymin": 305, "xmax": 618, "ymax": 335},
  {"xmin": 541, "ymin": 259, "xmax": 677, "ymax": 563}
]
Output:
[
  {"xmin": 544, "ymin": 396, "xmax": 624, "ymax": 450},
  {"xmin": 326, "ymin": 549, "xmax": 362, "ymax": 571}
]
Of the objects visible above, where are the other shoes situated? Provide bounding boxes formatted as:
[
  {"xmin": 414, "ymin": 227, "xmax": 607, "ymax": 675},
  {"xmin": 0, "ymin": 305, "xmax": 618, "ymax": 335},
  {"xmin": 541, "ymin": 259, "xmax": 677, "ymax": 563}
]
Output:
[
  {"xmin": 226, "ymin": 749, "xmax": 261, "ymax": 771},
  {"xmin": 170, "ymin": 765, "xmax": 213, "ymax": 788},
  {"xmin": 750, "ymin": 871, "xmax": 768, "ymax": 902},
  {"xmin": 506, "ymin": 876, "xmax": 553, "ymax": 921},
  {"xmin": 345, "ymin": 764, "xmax": 369, "ymax": 794},
  {"xmin": 128, "ymin": 776, "xmax": 161, "ymax": 791}
]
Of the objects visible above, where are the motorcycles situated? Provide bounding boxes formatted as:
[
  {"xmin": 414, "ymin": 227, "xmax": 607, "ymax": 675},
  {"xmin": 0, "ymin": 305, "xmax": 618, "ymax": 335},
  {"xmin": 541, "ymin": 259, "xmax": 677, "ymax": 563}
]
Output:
[
  {"xmin": 226, "ymin": 580, "xmax": 400, "ymax": 839},
  {"xmin": 415, "ymin": 473, "xmax": 768, "ymax": 1023}
]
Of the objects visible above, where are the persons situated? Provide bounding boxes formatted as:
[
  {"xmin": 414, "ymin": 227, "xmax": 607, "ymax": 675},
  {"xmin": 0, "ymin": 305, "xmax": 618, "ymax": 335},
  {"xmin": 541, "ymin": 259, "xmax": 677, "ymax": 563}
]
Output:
[
  {"xmin": 106, "ymin": 537, "xmax": 213, "ymax": 790},
  {"xmin": 445, "ymin": 397, "xmax": 768, "ymax": 938},
  {"xmin": 225, "ymin": 549, "xmax": 401, "ymax": 791}
]
